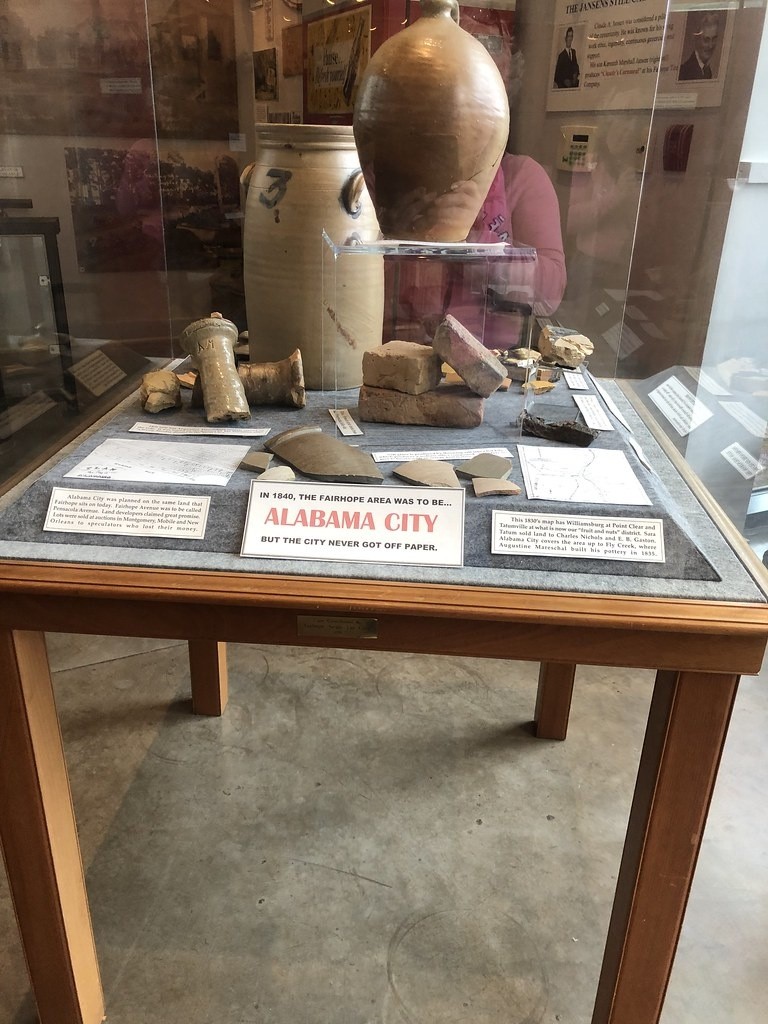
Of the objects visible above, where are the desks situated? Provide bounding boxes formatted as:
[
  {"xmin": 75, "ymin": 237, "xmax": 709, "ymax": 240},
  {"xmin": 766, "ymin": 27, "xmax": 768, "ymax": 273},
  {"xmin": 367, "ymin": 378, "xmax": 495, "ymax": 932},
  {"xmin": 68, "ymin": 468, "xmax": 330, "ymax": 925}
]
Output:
[{"xmin": 0, "ymin": 372, "xmax": 768, "ymax": 1023}]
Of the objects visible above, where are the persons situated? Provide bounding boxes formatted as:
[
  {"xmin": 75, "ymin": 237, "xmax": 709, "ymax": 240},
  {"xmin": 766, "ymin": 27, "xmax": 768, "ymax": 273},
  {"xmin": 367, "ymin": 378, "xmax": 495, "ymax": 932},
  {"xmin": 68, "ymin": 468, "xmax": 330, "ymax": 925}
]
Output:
[
  {"xmin": 382, "ymin": 148, "xmax": 569, "ymax": 369},
  {"xmin": 553, "ymin": 26, "xmax": 582, "ymax": 88},
  {"xmin": 679, "ymin": 11, "xmax": 718, "ymax": 81},
  {"xmin": 118, "ymin": 68, "xmax": 216, "ymax": 356}
]
